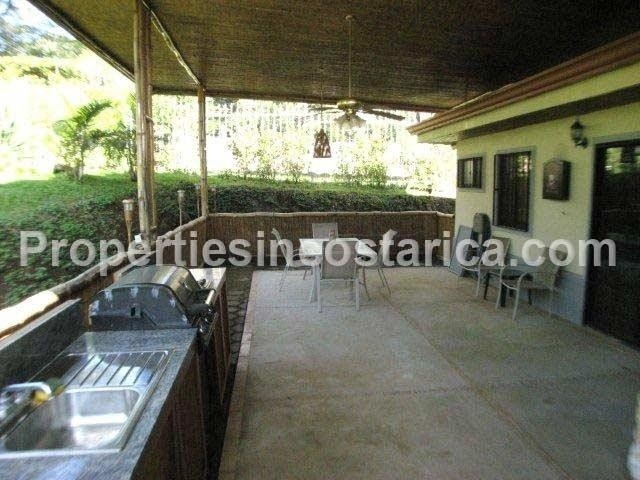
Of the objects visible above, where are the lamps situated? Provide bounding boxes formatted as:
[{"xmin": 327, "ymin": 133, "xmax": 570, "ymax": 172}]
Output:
[
  {"xmin": 312, "ymin": 81, "xmax": 331, "ymax": 158},
  {"xmin": 569, "ymin": 121, "xmax": 587, "ymax": 149},
  {"xmin": 334, "ymin": 109, "xmax": 365, "ymax": 128}
]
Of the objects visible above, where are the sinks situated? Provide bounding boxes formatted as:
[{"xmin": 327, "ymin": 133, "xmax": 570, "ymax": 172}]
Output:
[{"xmin": 0, "ymin": 385, "xmax": 139, "ymax": 454}]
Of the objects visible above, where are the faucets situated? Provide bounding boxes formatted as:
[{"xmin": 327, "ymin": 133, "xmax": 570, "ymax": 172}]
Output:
[{"xmin": 0, "ymin": 381, "xmax": 52, "ymax": 422}]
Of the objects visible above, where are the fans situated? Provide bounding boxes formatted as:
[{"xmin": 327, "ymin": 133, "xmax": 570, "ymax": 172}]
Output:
[{"xmin": 308, "ymin": 15, "xmax": 405, "ymax": 120}]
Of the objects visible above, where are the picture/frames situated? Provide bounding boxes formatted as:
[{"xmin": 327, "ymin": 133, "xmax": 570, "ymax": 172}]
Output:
[{"xmin": 542, "ymin": 158, "xmax": 570, "ymax": 200}]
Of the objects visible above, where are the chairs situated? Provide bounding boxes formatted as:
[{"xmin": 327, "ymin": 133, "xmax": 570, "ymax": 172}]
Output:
[
  {"xmin": 271, "ymin": 222, "xmax": 398, "ymax": 313},
  {"xmin": 458, "ymin": 236, "xmax": 568, "ymax": 321}
]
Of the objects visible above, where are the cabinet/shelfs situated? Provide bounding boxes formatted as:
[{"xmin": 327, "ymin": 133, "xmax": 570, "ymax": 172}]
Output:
[
  {"xmin": 132, "ymin": 340, "xmax": 220, "ymax": 480},
  {"xmin": 201, "ymin": 279, "xmax": 232, "ymax": 411}
]
